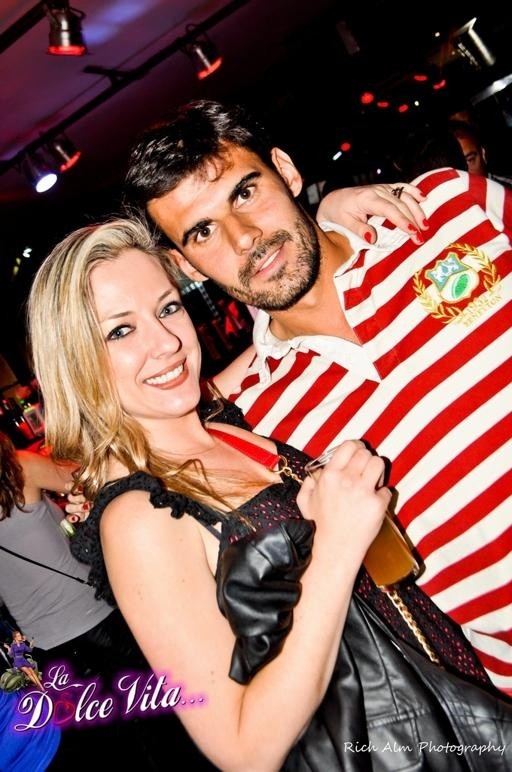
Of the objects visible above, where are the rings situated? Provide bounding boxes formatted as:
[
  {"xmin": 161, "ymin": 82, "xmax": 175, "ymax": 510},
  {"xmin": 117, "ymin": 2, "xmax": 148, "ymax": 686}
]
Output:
[{"xmin": 391, "ymin": 186, "xmax": 402, "ymax": 198}]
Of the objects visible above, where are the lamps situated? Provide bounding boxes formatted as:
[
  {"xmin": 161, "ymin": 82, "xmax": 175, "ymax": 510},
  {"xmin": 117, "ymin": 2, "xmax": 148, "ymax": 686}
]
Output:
[
  {"xmin": 20, "ymin": 153, "xmax": 58, "ymax": 194},
  {"xmin": 46, "ymin": 8, "xmax": 87, "ymax": 58},
  {"xmin": 188, "ymin": 39, "xmax": 225, "ymax": 81},
  {"xmin": 46, "ymin": 135, "xmax": 81, "ymax": 173}
]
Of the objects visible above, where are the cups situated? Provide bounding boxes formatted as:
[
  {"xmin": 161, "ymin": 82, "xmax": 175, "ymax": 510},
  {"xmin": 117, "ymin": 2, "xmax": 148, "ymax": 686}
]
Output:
[{"xmin": 306, "ymin": 445, "xmax": 424, "ymax": 592}]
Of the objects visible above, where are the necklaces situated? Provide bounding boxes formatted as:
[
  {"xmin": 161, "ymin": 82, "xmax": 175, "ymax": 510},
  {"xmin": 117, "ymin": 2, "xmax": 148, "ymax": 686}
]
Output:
[{"xmin": 153, "ymin": 441, "xmax": 221, "ymax": 457}]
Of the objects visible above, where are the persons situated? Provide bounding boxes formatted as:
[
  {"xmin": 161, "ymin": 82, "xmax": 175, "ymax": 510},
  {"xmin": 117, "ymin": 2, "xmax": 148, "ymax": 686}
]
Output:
[
  {"xmin": 391, "ymin": 107, "xmax": 490, "ymax": 182},
  {"xmin": 1, "ymin": 433, "xmax": 153, "ymax": 770},
  {"xmin": 28, "ymin": 180, "xmax": 512, "ymax": 771},
  {"xmin": 114, "ymin": 102, "xmax": 512, "ymax": 700},
  {"xmin": 3, "ymin": 630, "xmax": 46, "ymax": 693}
]
